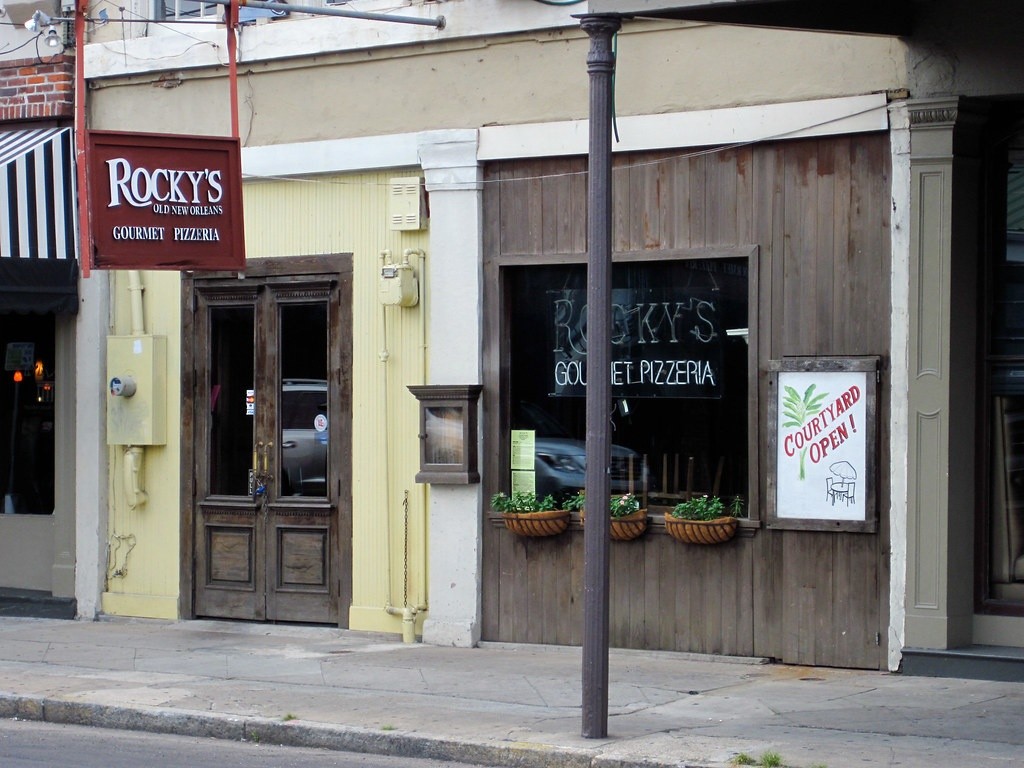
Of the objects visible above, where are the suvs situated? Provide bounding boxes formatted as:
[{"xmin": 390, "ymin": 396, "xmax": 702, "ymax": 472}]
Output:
[{"xmin": 282, "ymin": 378, "xmax": 329, "ymax": 490}]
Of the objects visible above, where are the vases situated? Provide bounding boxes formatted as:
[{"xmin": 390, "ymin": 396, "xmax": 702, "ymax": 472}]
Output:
[
  {"xmin": 580, "ymin": 509, "xmax": 647, "ymax": 541},
  {"xmin": 501, "ymin": 512, "xmax": 572, "ymax": 537},
  {"xmin": 665, "ymin": 513, "xmax": 738, "ymax": 545}
]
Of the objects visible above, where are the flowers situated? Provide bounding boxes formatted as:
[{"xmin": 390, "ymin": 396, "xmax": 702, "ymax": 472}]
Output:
[
  {"xmin": 491, "ymin": 493, "xmax": 557, "ymax": 514},
  {"xmin": 672, "ymin": 494, "xmax": 724, "ymax": 520},
  {"xmin": 577, "ymin": 490, "xmax": 640, "ymax": 517}
]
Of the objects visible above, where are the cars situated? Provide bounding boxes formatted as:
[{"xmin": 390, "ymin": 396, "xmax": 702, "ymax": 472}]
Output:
[{"xmin": 511, "ymin": 399, "xmax": 651, "ymax": 504}]
